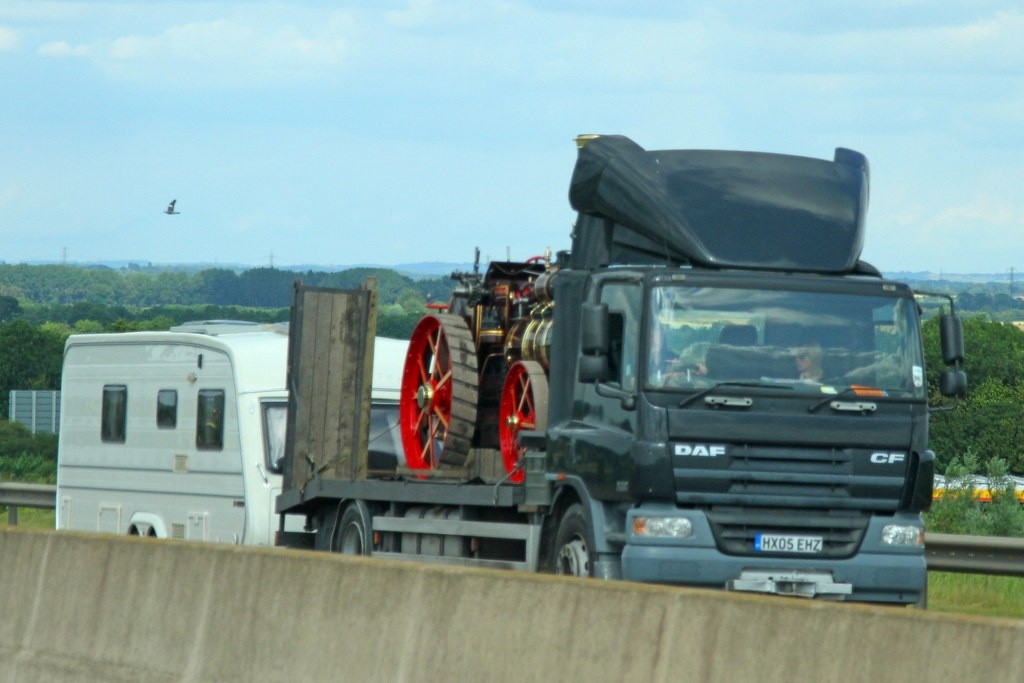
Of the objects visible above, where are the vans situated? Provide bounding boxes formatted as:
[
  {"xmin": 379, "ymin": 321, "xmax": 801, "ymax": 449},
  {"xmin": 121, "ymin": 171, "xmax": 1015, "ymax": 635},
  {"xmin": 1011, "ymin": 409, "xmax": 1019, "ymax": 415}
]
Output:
[{"xmin": 57, "ymin": 318, "xmax": 441, "ymax": 551}]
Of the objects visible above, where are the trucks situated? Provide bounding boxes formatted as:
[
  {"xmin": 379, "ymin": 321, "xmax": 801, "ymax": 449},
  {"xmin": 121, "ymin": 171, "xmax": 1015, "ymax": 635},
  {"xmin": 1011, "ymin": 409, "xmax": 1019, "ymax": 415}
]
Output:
[{"xmin": 275, "ymin": 133, "xmax": 970, "ymax": 609}]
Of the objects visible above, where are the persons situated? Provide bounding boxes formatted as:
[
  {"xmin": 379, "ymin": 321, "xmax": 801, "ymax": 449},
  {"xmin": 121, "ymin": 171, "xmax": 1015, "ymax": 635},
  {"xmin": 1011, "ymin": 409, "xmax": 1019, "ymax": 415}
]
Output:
[
  {"xmin": 646, "ymin": 319, "xmax": 708, "ymax": 380},
  {"xmin": 789, "ymin": 339, "xmax": 843, "ymax": 387}
]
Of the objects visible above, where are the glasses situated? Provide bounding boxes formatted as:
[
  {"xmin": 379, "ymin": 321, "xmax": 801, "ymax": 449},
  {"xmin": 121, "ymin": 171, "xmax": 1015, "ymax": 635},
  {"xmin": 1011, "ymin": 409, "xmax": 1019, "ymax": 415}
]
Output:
[{"xmin": 795, "ymin": 352, "xmax": 809, "ymax": 360}]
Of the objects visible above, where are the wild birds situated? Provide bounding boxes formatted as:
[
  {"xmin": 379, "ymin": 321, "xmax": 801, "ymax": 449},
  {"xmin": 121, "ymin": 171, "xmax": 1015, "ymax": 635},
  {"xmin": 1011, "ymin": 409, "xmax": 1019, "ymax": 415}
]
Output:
[{"xmin": 164, "ymin": 200, "xmax": 180, "ymax": 214}]
